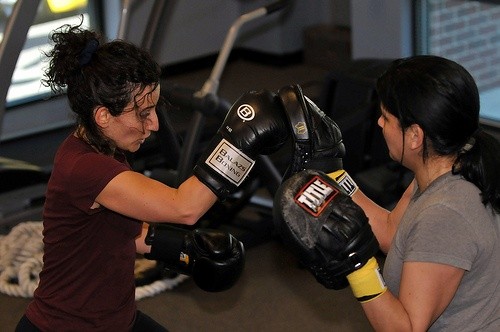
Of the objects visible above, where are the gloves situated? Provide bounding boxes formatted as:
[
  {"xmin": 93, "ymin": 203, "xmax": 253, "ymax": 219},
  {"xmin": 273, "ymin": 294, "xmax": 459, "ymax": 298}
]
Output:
[
  {"xmin": 144, "ymin": 223, "xmax": 246, "ymax": 293},
  {"xmin": 273, "ymin": 170, "xmax": 380, "ymax": 290},
  {"xmin": 192, "ymin": 79, "xmax": 290, "ymax": 202},
  {"xmin": 272, "ymin": 82, "xmax": 347, "ymax": 174}
]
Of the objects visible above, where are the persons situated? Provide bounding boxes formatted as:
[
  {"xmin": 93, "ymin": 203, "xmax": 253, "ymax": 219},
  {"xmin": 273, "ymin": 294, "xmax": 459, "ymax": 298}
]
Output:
[
  {"xmin": 274, "ymin": 56, "xmax": 500, "ymax": 332},
  {"xmin": 17, "ymin": 31, "xmax": 289, "ymax": 332}
]
